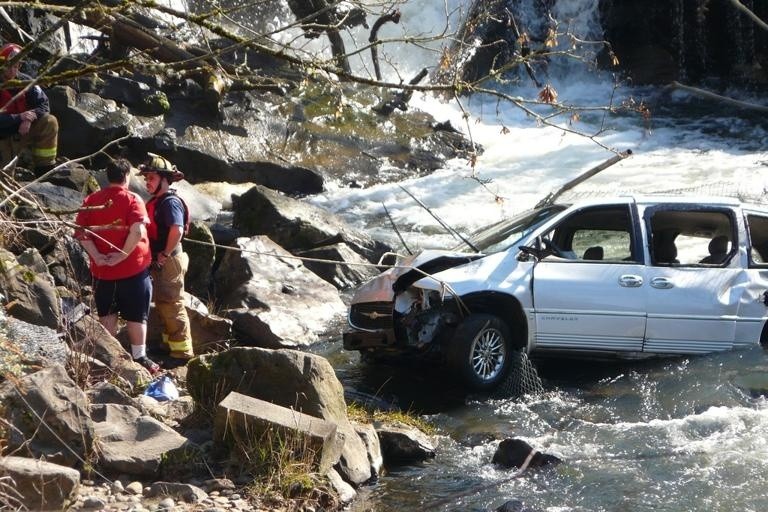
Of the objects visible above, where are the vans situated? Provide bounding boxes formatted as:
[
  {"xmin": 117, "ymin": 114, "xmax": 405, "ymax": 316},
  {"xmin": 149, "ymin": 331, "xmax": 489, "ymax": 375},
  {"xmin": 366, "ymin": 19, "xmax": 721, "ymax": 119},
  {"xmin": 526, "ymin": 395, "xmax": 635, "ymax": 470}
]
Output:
[{"xmin": 338, "ymin": 191, "xmax": 768, "ymax": 386}]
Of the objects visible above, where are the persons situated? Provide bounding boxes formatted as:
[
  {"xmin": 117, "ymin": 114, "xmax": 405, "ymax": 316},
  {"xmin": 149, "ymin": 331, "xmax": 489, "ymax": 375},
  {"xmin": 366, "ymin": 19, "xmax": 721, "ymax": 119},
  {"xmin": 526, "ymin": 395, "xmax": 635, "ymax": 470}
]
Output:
[
  {"xmin": 139, "ymin": 156, "xmax": 194, "ymax": 370},
  {"xmin": 75, "ymin": 158, "xmax": 160, "ymax": 374},
  {"xmin": 0, "ymin": 43, "xmax": 60, "ymax": 178}
]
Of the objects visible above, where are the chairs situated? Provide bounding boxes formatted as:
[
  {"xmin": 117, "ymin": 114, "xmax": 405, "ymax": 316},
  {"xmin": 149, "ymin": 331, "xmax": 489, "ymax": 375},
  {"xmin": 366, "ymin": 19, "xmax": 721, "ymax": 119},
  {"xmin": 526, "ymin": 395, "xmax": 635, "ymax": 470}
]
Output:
[
  {"xmin": 581, "ymin": 245, "xmax": 603, "ymax": 260},
  {"xmin": 696, "ymin": 235, "xmax": 729, "ymax": 264}
]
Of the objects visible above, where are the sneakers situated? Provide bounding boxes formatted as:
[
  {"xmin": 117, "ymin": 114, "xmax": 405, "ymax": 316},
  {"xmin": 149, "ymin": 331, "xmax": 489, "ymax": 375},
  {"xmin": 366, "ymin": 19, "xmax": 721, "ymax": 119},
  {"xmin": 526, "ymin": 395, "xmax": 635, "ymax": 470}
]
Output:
[
  {"xmin": 150, "ymin": 347, "xmax": 191, "ymax": 369},
  {"xmin": 36, "ymin": 165, "xmax": 72, "ymax": 176},
  {"xmin": 134, "ymin": 356, "xmax": 165, "ymax": 373}
]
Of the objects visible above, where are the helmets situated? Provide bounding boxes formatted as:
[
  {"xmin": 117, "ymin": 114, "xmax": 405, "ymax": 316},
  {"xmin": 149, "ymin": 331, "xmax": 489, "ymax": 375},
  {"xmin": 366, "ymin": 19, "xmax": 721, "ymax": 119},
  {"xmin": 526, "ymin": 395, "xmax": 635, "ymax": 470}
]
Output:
[
  {"xmin": 135, "ymin": 156, "xmax": 185, "ymax": 181},
  {"xmin": 1, "ymin": 43, "xmax": 25, "ymax": 69}
]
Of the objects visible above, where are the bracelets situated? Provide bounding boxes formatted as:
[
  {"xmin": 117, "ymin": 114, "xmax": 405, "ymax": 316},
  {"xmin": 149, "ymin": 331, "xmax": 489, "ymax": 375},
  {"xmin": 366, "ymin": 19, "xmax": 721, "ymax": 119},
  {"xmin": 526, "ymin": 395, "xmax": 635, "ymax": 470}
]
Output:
[{"xmin": 161, "ymin": 250, "xmax": 170, "ymax": 258}]
